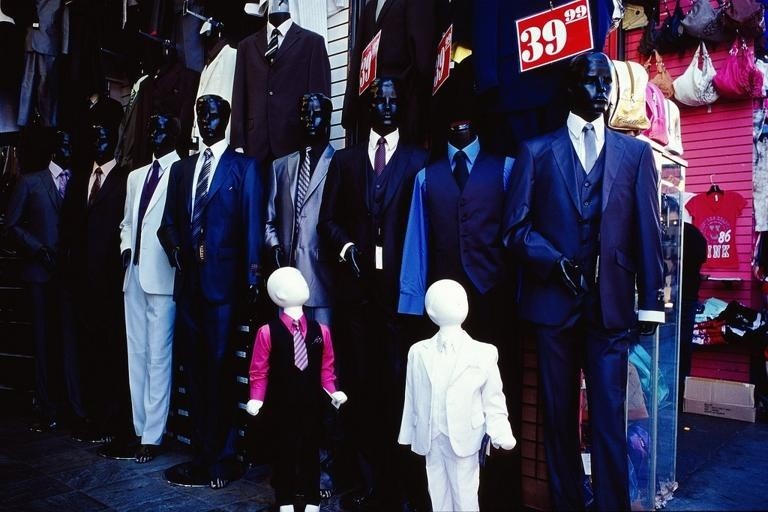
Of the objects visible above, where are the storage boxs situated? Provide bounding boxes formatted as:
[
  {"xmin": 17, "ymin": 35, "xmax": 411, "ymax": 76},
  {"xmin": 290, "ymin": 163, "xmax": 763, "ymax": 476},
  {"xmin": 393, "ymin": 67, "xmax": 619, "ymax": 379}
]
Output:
[{"xmin": 682, "ymin": 376, "xmax": 757, "ymax": 423}]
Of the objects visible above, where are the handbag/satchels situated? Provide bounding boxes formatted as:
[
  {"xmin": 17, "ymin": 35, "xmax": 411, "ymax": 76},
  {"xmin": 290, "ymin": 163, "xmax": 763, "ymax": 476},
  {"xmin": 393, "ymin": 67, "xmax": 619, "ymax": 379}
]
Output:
[{"xmin": 608, "ymin": 0, "xmax": 768, "ymax": 157}]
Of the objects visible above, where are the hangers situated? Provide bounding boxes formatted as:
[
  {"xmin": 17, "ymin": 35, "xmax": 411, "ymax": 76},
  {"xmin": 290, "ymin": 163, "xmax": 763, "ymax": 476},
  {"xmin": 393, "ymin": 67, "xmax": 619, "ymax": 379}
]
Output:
[{"xmin": 707, "ymin": 172, "xmax": 724, "ymax": 196}]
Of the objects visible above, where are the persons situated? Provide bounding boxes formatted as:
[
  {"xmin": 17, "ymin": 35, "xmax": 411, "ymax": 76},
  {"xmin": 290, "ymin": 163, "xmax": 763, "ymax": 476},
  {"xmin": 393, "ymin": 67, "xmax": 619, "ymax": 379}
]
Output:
[
  {"xmin": 10, "ymin": 14, "xmax": 665, "ymax": 511},
  {"xmin": 658, "ymin": 196, "xmax": 708, "ymax": 427}
]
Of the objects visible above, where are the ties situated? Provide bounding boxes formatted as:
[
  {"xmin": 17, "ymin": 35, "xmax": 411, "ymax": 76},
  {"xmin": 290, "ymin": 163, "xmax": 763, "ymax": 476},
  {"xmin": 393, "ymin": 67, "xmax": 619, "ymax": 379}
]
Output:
[
  {"xmin": 293, "ymin": 320, "xmax": 309, "ymax": 372},
  {"xmin": 58, "ymin": 172, "xmax": 68, "ymax": 197},
  {"xmin": 583, "ymin": 124, "xmax": 596, "ymax": 173},
  {"xmin": 88, "ymin": 168, "xmax": 103, "ymax": 206},
  {"xmin": 454, "ymin": 152, "xmax": 469, "ymax": 189},
  {"xmin": 128, "ymin": 90, "xmax": 136, "ymax": 113},
  {"xmin": 191, "ymin": 148, "xmax": 213, "ymax": 251},
  {"xmin": 373, "ymin": 138, "xmax": 387, "ymax": 176},
  {"xmin": 133, "ymin": 160, "xmax": 160, "ymax": 266},
  {"xmin": 264, "ymin": 29, "xmax": 281, "ymax": 65},
  {"xmin": 295, "ymin": 147, "xmax": 312, "ymax": 234}
]
